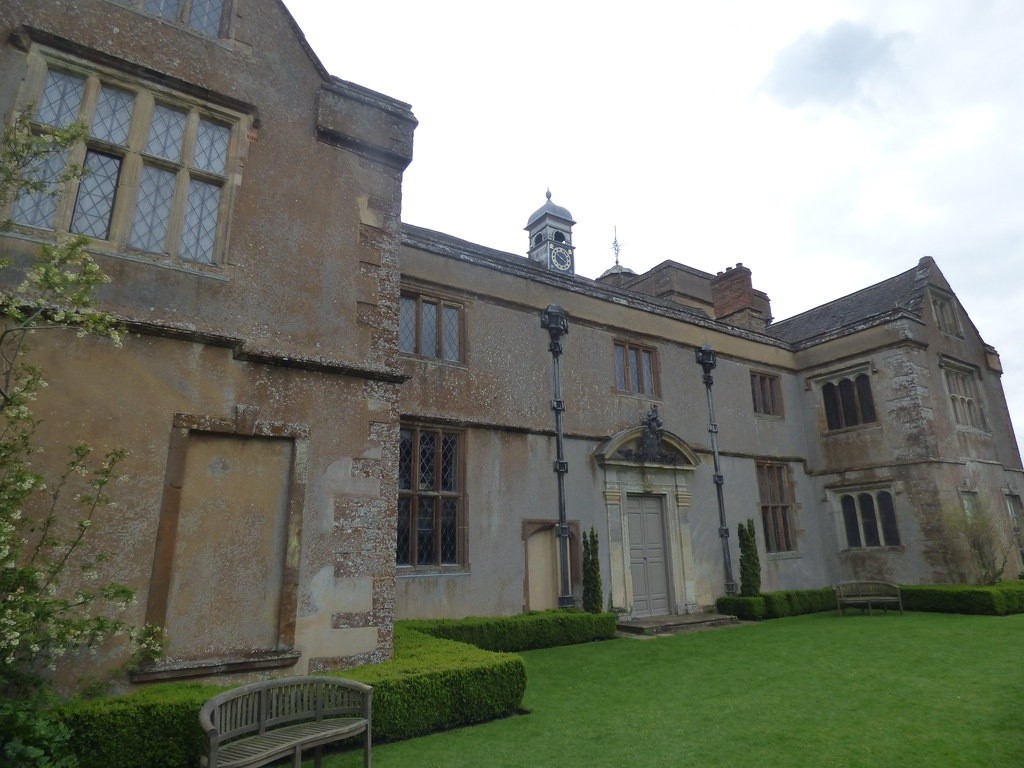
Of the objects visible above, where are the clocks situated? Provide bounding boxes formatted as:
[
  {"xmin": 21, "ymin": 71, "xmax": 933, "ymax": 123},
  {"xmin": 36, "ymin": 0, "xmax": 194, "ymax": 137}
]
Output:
[{"xmin": 548, "ymin": 238, "xmax": 576, "ymax": 277}]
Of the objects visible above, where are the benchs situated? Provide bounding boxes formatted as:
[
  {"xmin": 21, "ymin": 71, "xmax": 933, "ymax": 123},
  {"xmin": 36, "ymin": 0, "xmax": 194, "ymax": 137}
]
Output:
[
  {"xmin": 835, "ymin": 580, "xmax": 905, "ymax": 618},
  {"xmin": 199, "ymin": 676, "xmax": 374, "ymax": 768}
]
